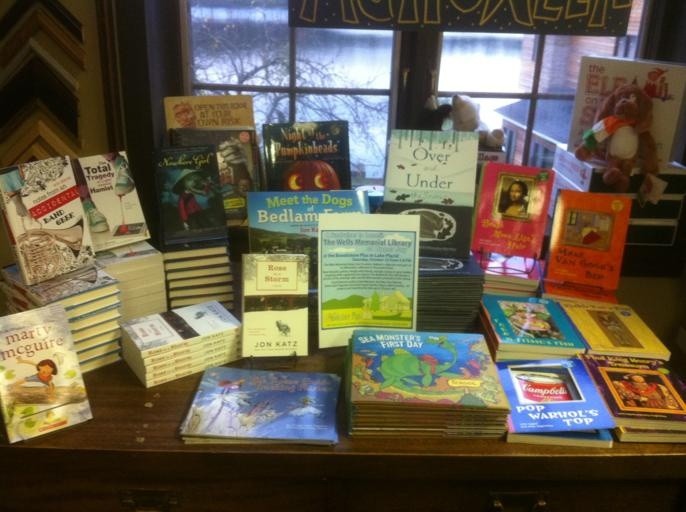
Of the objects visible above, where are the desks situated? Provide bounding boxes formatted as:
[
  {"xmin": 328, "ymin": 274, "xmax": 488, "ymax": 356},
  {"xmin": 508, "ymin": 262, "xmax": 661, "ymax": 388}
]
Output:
[{"xmin": 1, "ymin": 312, "xmax": 686, "ymax": 511}]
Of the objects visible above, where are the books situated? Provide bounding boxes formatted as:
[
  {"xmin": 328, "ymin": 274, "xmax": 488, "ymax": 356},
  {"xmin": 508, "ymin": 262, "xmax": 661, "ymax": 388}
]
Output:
[
  {"xmin": 0, "ymin": 149, "xmax": 168, "ymax": 444},
  {"xmin": 118, "ymin": 94, "xmax": 352, "ymax": 448},
  {"xmin": 544, "ymin": 54, "xmax": 686, "ymax": 247},
  {"xmin": 471, "ymin": 161, "xmax": 686, "ymax": 447},
  {"xmin": 317, "ymin": 126, "xmax": 512, "ymax": 440}
]
